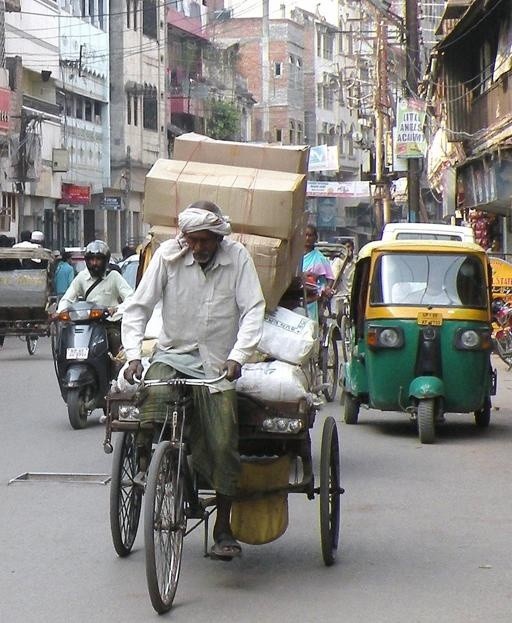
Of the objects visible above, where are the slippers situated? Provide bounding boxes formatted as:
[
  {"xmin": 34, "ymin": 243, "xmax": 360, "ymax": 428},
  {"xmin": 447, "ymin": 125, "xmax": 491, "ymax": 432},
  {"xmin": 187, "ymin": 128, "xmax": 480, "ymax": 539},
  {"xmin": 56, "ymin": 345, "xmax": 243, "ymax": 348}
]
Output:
[
  {"xmin": 133, "ymin": 471, "xmax": 145, "ymax": 486},
  {"xmin": 211, "ymin": 540, "xmax": 242, "ymax": 556}
]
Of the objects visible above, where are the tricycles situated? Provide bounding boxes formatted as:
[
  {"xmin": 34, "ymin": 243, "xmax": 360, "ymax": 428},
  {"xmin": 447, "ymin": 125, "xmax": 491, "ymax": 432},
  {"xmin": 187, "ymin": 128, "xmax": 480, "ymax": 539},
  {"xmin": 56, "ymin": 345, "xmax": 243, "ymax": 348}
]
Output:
[
  {"xmin": 1, "ymin": 247, "xmax": 60, "ymax": 356},
  {"xmin": 106, "ymin": 366, "xmax": 348, "ymax": 616},
  {"xmin": 304, "ymin": 241, "xmax": 351, "ymax": 402}
]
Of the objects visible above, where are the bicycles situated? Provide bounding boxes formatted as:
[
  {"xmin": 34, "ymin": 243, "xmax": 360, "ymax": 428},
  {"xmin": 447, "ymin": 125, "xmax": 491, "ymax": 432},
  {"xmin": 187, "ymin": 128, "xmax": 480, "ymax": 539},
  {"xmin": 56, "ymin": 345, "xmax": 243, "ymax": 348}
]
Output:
[{"xmin": 334, "ymin": 294, "xmax": 353, "ymax": 364}]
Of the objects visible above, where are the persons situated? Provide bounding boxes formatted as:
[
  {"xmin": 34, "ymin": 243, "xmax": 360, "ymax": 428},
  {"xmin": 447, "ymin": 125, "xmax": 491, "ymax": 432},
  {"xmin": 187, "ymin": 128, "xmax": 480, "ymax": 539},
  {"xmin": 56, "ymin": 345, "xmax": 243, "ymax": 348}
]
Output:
[
  {"xmin": 328, "ymin": 236, "xmax": 358, "ymax": 328},
  {"xmin": 0, "ymin": 226, "xmax": 135, "ymax": 359},
  {"xmin": 114, "ymin": 200, "xmax": 267, "ymax": 561},
  {"xmin": 299, "ymin": 225, "xmax": 333, "ymax": 336}
]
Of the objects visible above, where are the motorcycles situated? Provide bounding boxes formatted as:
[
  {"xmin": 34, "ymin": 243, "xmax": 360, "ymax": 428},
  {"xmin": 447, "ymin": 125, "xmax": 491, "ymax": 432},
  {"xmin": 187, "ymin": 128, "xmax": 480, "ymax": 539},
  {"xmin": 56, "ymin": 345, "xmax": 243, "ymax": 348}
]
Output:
[
  {"xmin": 492, "ymin": 287, "xmax": 512, "ymax": 371},
  {"xmin": 49, "ymin": 304, "xmax": 121, "ymax": 429},
  {"xmin": 338, "ymin": 238, "xmax": 498, "ymax": 444}
]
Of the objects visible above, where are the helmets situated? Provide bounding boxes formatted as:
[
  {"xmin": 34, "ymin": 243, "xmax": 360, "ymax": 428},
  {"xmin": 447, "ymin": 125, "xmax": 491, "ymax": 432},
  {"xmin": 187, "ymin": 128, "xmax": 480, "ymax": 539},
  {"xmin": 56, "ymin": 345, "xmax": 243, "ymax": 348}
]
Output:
[{"xmin": 84, "ymin": 240, "xmax": 111, "ymax": 277}]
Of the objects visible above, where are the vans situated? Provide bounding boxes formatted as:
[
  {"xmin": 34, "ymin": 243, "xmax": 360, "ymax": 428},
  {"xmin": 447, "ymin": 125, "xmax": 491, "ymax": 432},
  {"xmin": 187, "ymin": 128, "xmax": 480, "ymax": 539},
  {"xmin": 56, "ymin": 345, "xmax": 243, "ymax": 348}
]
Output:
[{"xmin": 381, "ymin": 221, "xmax": 476, "ymax": 248}]
